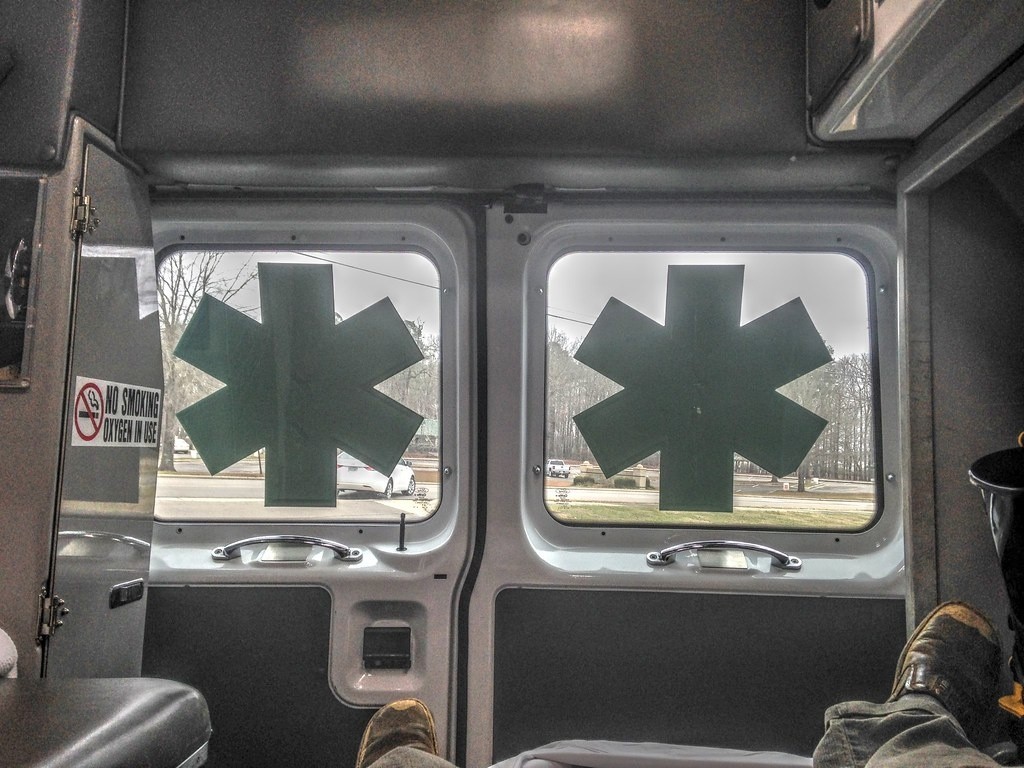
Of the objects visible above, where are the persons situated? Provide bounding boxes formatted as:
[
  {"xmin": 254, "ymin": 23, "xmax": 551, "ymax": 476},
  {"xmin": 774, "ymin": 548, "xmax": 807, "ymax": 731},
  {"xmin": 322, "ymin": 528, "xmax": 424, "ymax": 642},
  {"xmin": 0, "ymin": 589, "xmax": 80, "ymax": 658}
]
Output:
[{"xmin": 355, "ymin": 601, "xmax": 1003, "ymax": 768}]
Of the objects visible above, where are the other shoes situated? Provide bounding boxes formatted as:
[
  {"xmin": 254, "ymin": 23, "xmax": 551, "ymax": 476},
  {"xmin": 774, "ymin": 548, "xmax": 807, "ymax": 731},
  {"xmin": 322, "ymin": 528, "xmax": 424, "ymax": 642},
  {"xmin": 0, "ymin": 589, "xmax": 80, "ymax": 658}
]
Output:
[
  {"xmin": 356, "ymin": 700, "xmax": 438, "ymax": 768},
  {"xmin": 894, "ymin": 601, "xmax": 1004, "ymax": 739}
]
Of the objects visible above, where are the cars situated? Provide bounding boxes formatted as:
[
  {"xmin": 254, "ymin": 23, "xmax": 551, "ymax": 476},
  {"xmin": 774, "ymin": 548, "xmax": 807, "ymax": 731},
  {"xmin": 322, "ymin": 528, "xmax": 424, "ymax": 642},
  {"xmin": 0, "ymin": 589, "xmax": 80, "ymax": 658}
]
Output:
[
  {"xmin": 174, "ymin": 439, "xmax": 189, "ymax": 454},
  {"xmin": 336, "ymin": 450, "xmax": 416, "ymax": 499}
]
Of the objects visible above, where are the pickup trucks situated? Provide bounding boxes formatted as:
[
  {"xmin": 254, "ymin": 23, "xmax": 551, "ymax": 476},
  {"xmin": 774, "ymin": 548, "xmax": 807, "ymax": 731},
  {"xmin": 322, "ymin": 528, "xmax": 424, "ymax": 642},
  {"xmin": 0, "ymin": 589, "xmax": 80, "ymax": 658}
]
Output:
[{"xmin": 545, "ymin": 459, "xmax": 571, "ymax": 478}]
